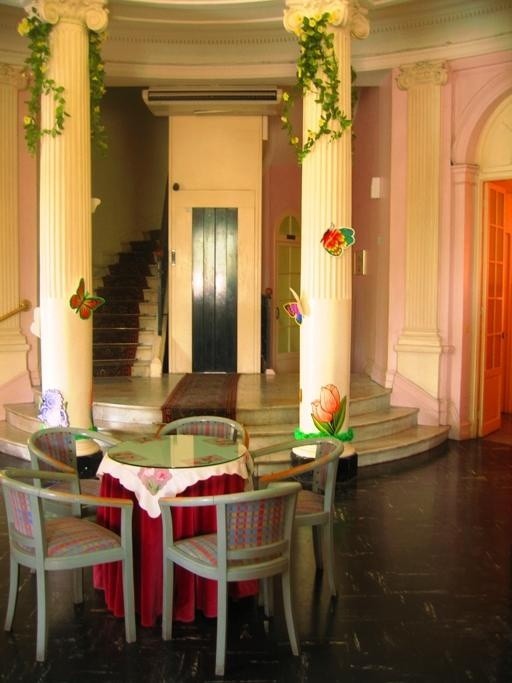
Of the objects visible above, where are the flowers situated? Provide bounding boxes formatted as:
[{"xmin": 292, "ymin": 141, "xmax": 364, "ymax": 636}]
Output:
[
  {"xmin": 275, "ymin": 1, "xmax": 351, "ymax": 167},
  {"xmin": 309, "ymin": 384, "xmax": 350, "ymax": 435},
  {"xmin": 88, "ymin": 26, "xmax": 112, "ymax": 154},
  {"xmin": 18, "ymin": 7, "xmax": 72, "ymax": 157}
]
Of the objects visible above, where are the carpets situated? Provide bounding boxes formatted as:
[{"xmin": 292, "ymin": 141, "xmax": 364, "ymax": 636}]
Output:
[{"xmin": 159, "ymin": 370, "xmax": 243, "ymax": 438}]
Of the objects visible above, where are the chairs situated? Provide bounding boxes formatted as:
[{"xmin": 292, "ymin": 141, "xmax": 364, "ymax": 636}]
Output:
[
  {"xmin": 250, "ymin": 437, "xmax": 344, "ymax": 618},
  {"xmin": 0, "ymin": 467, "xmax": 138, "ymax": 662},
  {"xmin": 159, "ymin": 482, "xmax": 301, "ymax": 677},
  {"xmin": 160, "ymin": 416, "xmax": 250, "ymax": 452},
  {"xmin": 28, "ymin": 427, "xmax": 117, "ymax": 604}
]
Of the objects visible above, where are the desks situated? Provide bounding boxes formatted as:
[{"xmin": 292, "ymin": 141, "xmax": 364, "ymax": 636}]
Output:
[{"xmin": 94, "ymin": 434, "xmax": 260, "ymax": 628}]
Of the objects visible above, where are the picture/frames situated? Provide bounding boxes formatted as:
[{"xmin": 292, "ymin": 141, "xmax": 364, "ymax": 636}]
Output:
[{"xmin": 353, "ymin": 249, "xmax": 366, "ymax": 276}]
